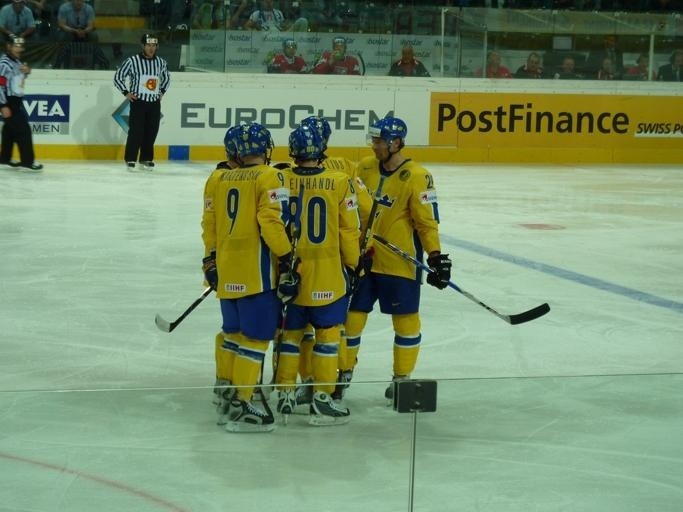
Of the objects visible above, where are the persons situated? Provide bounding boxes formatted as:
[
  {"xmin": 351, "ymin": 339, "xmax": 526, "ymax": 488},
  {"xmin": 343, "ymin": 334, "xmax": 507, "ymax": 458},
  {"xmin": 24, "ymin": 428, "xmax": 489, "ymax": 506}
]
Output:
[
  {"xmin": 1, "ymin": 0, "xmax": 189, "ymax": 72},
  {"xmin": 111, "ymin": 33, "xmax": 169, "ymax": 168},
  {"xmin": 0, "ymin": 31, "xmax": 44, "ymax": 169},
  {"xmin": 189, "ymin": 1, "xmax": 460, "ymax": 36},
  {"xmin": 388, "ymin": 44, "xmax": 429, "ymax": 76},
  {"xmin": 313, "ymin": 35, "xmax": 366, "ymax": 75},
  {"xmin": 331, "ymin": 115, "xmax": 452, "ymax": 412},
  {"xmin": 471, "ymin": 0, "xmax": 682, "ymax": 81},
  {"xmin": 265, "ymin": 38, "xmax": 308, "ymax": 74}
]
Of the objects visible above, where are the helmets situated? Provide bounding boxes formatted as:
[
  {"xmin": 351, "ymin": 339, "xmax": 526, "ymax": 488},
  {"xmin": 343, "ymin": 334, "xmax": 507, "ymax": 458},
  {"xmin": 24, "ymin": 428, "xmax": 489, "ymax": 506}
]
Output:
[
  {"xmin": 287, "ymin": 115, "xmax": 331, "ymax": 163},
  {"xmin": 141, "ymin": 34, "xmax": 158, "ymax": 46},
  {"xmin": 222, "ymin": 123, "xmax": 274, "ymax": 160},
  {"xmin": 282, "ymin": 38, "xmax": 297, "ymax": 50},
  {"xmin": 332, "ymin": 37, "xmax": 345, "ymax": 46},
  {"xmin": 6, "ymin": 34, "xmax": 24, "ymax": 47},
  {"xmin": 366, "ymin": 117, "xmax": 407, "ymax": 148}
]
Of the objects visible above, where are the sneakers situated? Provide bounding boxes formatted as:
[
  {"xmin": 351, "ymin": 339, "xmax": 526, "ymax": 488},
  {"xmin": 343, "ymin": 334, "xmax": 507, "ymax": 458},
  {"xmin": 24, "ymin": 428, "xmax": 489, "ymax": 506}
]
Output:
[
  {"xmin": 20, "ymin": 162, "xmax": 43, "ymax": 170},
  {"xmin": 124, "ymin": 160, "xmax": 135, "ymax": 167},
  {"xmin": 138, "ymin": 161, "xmax": 154, "ymax": 167},
  {"xmin": 0, "ymin": 160, "xmax": 19, "ymax": 167}
]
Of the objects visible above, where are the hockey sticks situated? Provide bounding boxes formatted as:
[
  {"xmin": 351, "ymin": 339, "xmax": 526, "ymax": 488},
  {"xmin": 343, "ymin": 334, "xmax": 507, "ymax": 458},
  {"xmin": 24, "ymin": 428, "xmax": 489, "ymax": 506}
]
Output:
[
  {"xmin": 372, "ymin": 234, "xmax": 551, "ymax": 326},
  {"xmin": 257, "ymin": 357, "xmax": 274, "ymax": 418},
  {"xmin": 154, "ymin": 286, "xmax": 215, "ymax": 333},
  {"xmin": 261, "ymin": 231, "xmax": 306, "ymax": 395}
]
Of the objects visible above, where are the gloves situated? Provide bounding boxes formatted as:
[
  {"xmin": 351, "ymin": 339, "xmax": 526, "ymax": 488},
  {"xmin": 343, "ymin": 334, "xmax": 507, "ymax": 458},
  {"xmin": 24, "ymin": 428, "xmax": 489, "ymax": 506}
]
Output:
[
  {"xmin": 276, "ymin": 250, "xmax": 302, "ymax": 305},
  {"xmin": 426, "ymin": 251, "xmax": 452, "ymax": 290},
  {"xmin": 343, "ymin": 256, "xmax": 367, "ymax": 280},
  {"xmin": 200, "ymin": 255, "xmax": 218, "ymax": 292}
]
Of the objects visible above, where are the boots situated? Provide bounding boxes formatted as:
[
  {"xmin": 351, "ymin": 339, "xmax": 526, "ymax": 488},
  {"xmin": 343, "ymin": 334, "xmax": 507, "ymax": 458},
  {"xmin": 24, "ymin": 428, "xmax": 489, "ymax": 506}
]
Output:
[
  {"xmin": 385, "ymin": 374, "xmax": 410, "ymax": 399},
  {"xmin": 294, "ymin": 369, "xmax": 353, "ymax": 406},
  {"xmin": 276, "ymin": 389, "xmax": 295, "ymax": 414},
  {"xmin": 310, "ymin": 391, "xmax": 350, "ymax": 416},
  {"xmin": 213, "ymin": 375, "xmax": 273, "ymax": 424}
]
